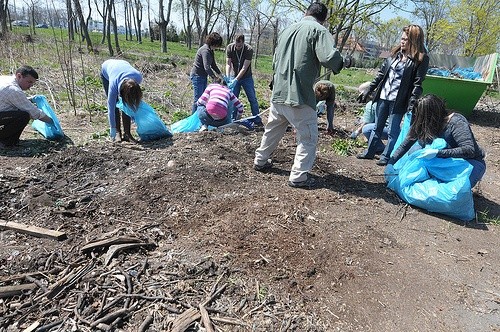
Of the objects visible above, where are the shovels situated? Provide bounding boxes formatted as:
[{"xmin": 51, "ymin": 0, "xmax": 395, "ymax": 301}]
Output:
[{"xmin": 233, "ymin": 69, "xmax": 333, "ymax": 123}]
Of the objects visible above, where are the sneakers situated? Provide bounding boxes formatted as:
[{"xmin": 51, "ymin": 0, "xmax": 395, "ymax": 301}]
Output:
[
  {"xmin": 255, "ymin": 121, "xmax": 264, "ymax": 127},
  {"xmin": 253, "ymin": 159, "xmax": 273, "ymax": 170},
  {"xmin": 199, "ymin": 124, "xmax": 208, "ymax": 132},
  {"xmin": 325, "ymin": 128, "xmax": 335, "ymax": 134},
  {"xmin": 288, "ymin": 175, "xmax": 317, "ymax": 187},
  {"xmin": 0, "ymin": 142, "xmax": 15, "ymax": 149}
]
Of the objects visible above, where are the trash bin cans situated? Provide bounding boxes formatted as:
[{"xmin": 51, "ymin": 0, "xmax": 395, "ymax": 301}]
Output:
[{"xmin": 419, "ymin": 52, "xmax": 499, "ymax": 116}]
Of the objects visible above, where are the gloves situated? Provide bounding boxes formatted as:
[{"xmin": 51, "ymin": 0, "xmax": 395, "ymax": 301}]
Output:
[
  {"xmin": 407, "ymin": 104, "xmax": 416, "ymax": 114},
  {"xmin": 416, "ymin": 149, "xmax": 439, "ymax": 160},
  {"xmin": 344, "ymin": 54, "xmax": 353, "ymax": 68},
  {"xmin": 215, "ymin": 73, "xmax": 238, "ymax": 89},
  {"xmin": 110, "ymin": 129, "xmax": 116, "ymax": 140},
  {"xmin": 350, "ymin": 130, "xmax": 360, "ymax": 139},
  {"xmin": 357, "ymin": 87, "xmax": 371, "ymax": 104},
  {"xmin": 317, "ymin": 105, "xmax": 327, "ymax": 114},
  {"xmin": 269, "ymin": 81, "xmax": 273, "ymax": 91},
  {"xmin": 364, "ymin": 136, "xmax": 370, "ymax": 143},
  {"xmin": 384, "ymin": 164, "xmax": 394, "ymax": 183}
]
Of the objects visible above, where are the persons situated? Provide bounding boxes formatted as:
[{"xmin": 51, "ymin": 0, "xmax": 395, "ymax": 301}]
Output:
[
  {"xmin": 389, "ymin": 94, "xmax": 486, "ymax": 188},
  {"xmin": 357, "ymin": 25, "xmax": 429, "ymax": 165},
  {"xmin": 313, "ymin": 79, "xmax": 336, "ymax": 134},
  {"xmin": 225, "ymin": 36, "xmax": 263, "ymax": 125},
  {"xmin": 0, "ymin": 65, "xmax": 53, "ymax": 150},
  {"xmin": 351, "ymin": 82, "xmax": 390, "ymax": 154},
  {"xmin": 195, "ymin": 78, "xmax": 244, "ymax": 133},
  {"xmin": 190, "ymin": 32, "xmax": 224, "ymax": 115},
  {"xmin": 254, "ymin": 3, "xmax": 344, "ymax": 187},
  {"xmin": 100, "ymin": 59, "xmax": 143, "ymax": 143}
]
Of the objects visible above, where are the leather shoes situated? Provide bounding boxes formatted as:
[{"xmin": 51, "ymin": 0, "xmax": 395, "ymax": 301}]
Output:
[
  {"xmin": 356, "ymin": 152, "xmax": 389, "ymax": 166},
  {"xmin": 123, "ymin": 133, "xmax": 137, "ymax": 143},
  {"xmin": 115, "ymin": 129, "xmax": 122, "ymax": 142}
]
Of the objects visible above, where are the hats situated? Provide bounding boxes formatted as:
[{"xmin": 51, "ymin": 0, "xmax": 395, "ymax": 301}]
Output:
[{"xmin": 358, "ymin": 81, "xmax": 377, "ymax": 96}]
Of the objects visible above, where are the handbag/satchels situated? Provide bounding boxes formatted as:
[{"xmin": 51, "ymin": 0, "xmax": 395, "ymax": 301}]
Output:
[
  {"xmin": 223, "ymin": 79, "xmax": 235, "ymax": 124},
  {"xmin": 385, "ymin": 110, "xmax": 476, "ymax": 222},
  {"xmin": 172, "ymin": 110, "xmax": 203, "ymax": 135},
  {"xmin": 32, "ymin": 96, "xmax": 65, "ymax": 140},
  {"xmin": 115, "ymin": 97, "xmax": 173, "ymax": 140}
]
